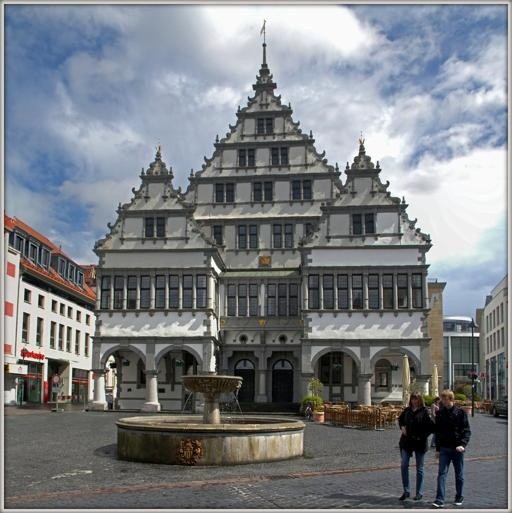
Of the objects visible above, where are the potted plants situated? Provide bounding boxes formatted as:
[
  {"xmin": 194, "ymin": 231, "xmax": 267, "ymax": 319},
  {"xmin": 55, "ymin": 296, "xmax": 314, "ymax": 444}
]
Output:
[
  {"xmin": 460, "ymin": 403, "xmax": 473, "ymax": 416},
  {"xmin": 311, "ymin": 407, "xmax": 325, "ymax": 423}
]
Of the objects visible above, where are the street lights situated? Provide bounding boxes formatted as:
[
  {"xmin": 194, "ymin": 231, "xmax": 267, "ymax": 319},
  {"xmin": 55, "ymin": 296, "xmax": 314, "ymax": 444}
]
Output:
[{"xmin": 469, "ymin": 318, "xmax": 478, "ymax": 416}]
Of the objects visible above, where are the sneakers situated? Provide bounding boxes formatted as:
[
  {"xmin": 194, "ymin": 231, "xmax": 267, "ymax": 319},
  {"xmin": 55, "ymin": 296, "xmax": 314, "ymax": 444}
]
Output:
[
  {"xmin": 432, "ymin": 500, "xmax": 444, "ymax": 509},
  {"xmin": 413, "ymin": 494, "xmax": 423, "ymax": 500},
  {"xmin": 453, "ymin": 494, "xmax": 464, "ymax": 506},
  {"xmin": 398, "ymin": 492, "xmax": 410, "ymax": 500}
]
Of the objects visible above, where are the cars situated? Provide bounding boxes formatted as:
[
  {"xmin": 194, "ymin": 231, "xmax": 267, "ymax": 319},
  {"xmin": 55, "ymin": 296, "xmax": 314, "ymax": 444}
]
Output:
[{"xmin": 492, "ymin": 396, "xmax": 508, "ymax": 417}]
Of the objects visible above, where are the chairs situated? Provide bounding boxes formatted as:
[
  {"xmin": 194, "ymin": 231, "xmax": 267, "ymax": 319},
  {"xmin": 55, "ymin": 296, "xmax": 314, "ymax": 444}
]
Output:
[
  {"xmin": 324, "ymin": 401, "xmax": 405, "ymax": 431},
  {"xmin": 473, "ymin": 399, "xmax": 493, "ymax": 416}
]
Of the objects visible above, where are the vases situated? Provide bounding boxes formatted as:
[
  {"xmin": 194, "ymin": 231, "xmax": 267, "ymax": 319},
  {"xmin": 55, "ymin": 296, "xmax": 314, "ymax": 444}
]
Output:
[{"xmin": 308, "ymin": 416, "xmax": 310, "ymax": 420}]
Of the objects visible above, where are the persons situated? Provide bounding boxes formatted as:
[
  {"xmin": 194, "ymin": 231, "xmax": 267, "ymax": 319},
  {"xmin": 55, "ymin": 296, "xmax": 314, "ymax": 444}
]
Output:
[
  {"xmin": 431, "ymin": 397, "xmax": 441, "ymax": 447},
  {"xmin": 399, "ymin": 391, "xmax": 430, "ymax": 500},
  {"xmin": 432, "ymin": 390, "xmax": 470, "ymax": 508}
]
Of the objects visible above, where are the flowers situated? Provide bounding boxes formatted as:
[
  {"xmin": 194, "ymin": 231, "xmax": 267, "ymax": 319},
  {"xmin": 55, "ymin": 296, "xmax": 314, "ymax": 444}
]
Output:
[{"xmin": 304, "ymin": 405, "xmax": 312, "ymax": 416}]
